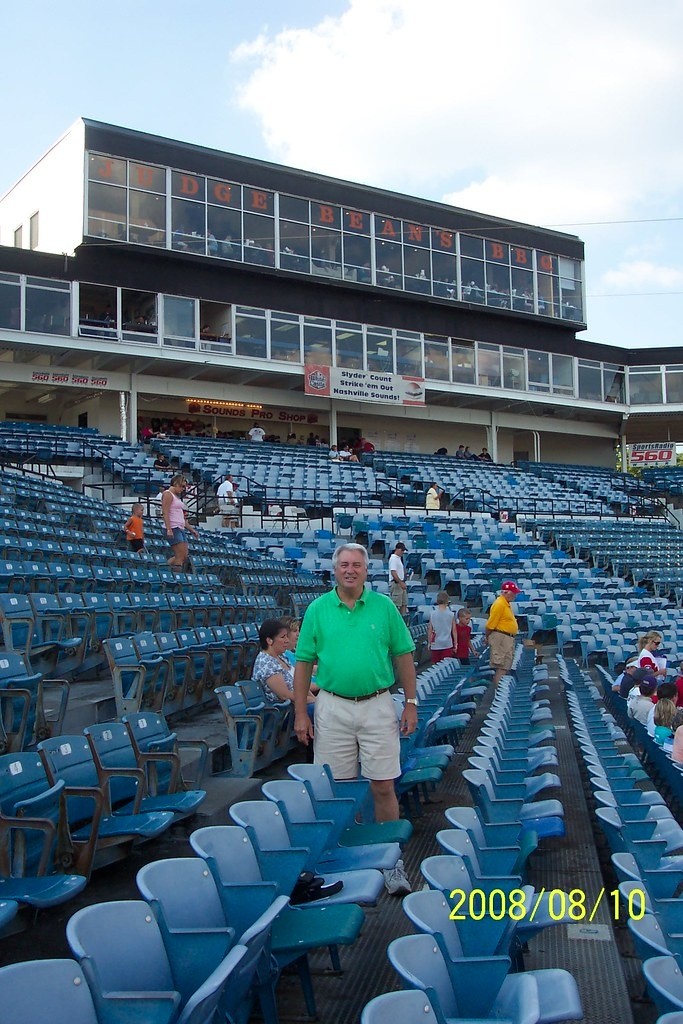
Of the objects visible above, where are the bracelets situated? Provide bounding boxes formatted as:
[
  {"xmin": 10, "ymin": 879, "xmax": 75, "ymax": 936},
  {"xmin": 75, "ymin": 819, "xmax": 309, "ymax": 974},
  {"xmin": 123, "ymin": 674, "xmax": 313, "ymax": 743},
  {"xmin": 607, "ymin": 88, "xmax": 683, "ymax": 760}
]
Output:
[{"xmin": 405, "ymin": 697, "xmax": 418, "ymax": 706}]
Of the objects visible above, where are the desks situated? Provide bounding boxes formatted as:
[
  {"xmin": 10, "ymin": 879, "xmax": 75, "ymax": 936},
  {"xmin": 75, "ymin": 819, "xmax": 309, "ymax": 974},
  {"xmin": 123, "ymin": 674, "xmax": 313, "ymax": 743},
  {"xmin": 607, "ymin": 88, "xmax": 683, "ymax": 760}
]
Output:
[
  {"xmin": 79, "ymin": 316, "xmax": 217, "ymax": 340},
  {"xmin": 128, "ymin": 225, "xmax": 546, "ymax": 312}
]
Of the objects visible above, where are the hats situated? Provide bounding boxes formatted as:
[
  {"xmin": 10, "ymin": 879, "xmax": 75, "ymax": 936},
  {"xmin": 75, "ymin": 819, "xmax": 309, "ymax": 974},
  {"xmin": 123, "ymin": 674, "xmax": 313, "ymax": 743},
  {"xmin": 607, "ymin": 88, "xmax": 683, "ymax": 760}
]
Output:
[
  {"xmin": 502, "ymin": 582, "xmax": 520, "ymax": 593},
  {"xmin": 641, "ymin": 675, "xmax": 658, "ymax": 688},
  {"xmin": 633, "ymin": 668, "xmax": 654, "ymax": 682},
  {"xmin": 396, "ymin": 543, "xmax": 408, "ymax": 551}
]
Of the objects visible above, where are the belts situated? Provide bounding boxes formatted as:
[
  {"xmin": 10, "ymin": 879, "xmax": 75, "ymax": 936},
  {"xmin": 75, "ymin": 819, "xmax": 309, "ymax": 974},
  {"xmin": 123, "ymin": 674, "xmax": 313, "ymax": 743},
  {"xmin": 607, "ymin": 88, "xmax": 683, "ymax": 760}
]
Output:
[
  {"xmin": 393, "ymin": 580, "xmax": 405, "ymax": 583},
  {"xmin": 493, "ymin": 629, "xmax": 514, "ymax": 637},
  {"xmin": 323, "ymin": 688, "xmax": 387, "ymax": 701}
]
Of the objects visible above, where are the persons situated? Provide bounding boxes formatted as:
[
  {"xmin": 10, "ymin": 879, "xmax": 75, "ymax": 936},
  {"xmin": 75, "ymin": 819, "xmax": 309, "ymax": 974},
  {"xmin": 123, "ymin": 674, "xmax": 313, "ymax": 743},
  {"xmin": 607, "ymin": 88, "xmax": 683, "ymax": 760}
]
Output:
[
  {"xmin": 427, "ymin": 592, "xmax": 457, "ymax": 664},
  {"xmin": 456, "ymin": 445, "xmax": 491, "ymax": 460},
  {"xmin": 425, "ymin": 482, "xmax": 443, "ymax": 509},
  {"xmin": 161, "ymin": 474, "xmax": 200, "ymax": 565},
  {"xmin": 387, "ymin": 543, "xmax": 411, "ymax": 616},
  {"xmin": 216, "ymin": 474, "xmax": 243, "ymax": 514},
  {"xmin": 251, "ymin": 619, "xmax": 317, "ymax": 724},
  {"xmin": 484, "ymin": 581, "xmax": 518, "ymax": 688},
  {"xmin": 612, "ymin": 630, "xmax": 683, "ymax": 763},
  {"xmin": 124, "ymin": 503, "xmax": 144, "ymax": 553},
  {"xmin": 451, "ymin": 608, "xmax": 477, "ymax": 665},
  {"xmin": 277, "ymin": 616, "xmax": 320, "ymax": 692},
  {"xmin": 139, "ymin": 422, "xmax": 170, "ymax": 440},
  {"xmin": 153, "ymin": 453, "xmax": 172, "ymax": 471},
  {"xmin": 154, "ymin": 485, "xmax": 165, "ymax": 526},
  {"xmin": 99, "ymin": 224, "xmax": 545, "ymax": 333},
  {"xmin": 328, "ymin": 437, "xmax": 374, "ymax": 462},
  {"xmin": 247, "ymin": 422, "xmax": 322, "ymax": 446},
  {"xmin": 294, "ymin": 542, "xmax": 418, "ymax": 896}
]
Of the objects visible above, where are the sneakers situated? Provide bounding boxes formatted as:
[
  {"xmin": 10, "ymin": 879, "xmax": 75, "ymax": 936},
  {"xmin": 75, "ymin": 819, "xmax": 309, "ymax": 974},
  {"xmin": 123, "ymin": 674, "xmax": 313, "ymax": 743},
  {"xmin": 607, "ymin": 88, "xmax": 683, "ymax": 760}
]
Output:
[{"xmin": 382, "ymin": 860, "xmax": 412, "ymax": 896}]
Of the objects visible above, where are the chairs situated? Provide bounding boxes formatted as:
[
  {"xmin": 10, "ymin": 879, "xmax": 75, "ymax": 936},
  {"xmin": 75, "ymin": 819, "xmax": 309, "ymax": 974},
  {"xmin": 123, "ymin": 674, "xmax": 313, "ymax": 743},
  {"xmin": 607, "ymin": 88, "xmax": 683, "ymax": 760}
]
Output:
[{"xmin": 0, "ymin": 419, "xmax": 683, "ymax": 1024}]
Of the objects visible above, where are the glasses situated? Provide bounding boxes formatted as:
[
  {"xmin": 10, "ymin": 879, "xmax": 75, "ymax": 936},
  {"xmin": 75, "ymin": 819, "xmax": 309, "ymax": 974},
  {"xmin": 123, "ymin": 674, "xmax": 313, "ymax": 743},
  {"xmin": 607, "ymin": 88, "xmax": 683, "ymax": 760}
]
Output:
[
  {"xmin": 402, "ymin": 549, "xmax": 404, "ymax": 551},
  {"xmin": 292, "ymin": 617, "xmax": 302, "ymax": 623},
  {"xmin": 652, "ymin": 641, "xmax": 660, "ymax": 646}
]
